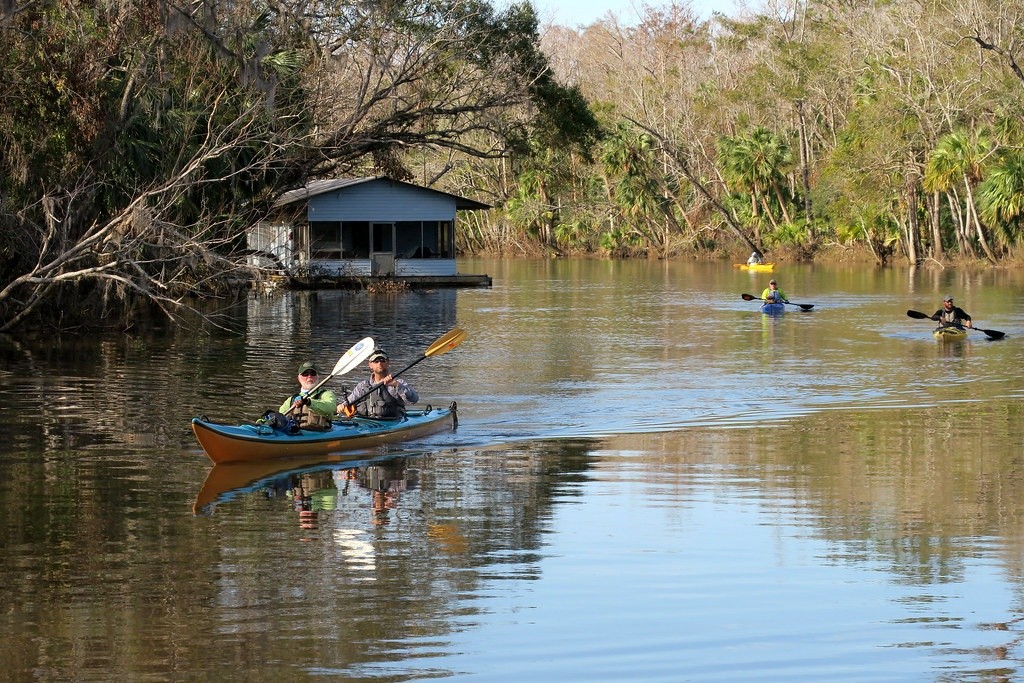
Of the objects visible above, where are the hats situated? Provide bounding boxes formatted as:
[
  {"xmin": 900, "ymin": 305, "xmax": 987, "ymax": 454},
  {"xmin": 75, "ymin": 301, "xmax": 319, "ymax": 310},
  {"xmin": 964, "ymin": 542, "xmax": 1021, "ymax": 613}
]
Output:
[
  {"xmin": 369, "ymin": 349, "xmax": 387, "ymax": 362},
  {"xmin": 298, "ymin": 361, "xmax": 318, "ymax": 374}
]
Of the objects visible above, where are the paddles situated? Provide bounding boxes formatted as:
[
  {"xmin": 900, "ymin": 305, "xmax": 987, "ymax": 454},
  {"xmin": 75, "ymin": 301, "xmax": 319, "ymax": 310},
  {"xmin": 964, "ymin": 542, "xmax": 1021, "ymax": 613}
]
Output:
[
  {"xmin": 907, "ymin": 308, "xmax": 1006, "ymax": 338},
  {"xmin": 282, "ymin": 336, "xmax": 376, "ymax": 416},
  {"xmin": 742, "ymin": 293, "xmax": 814, "ymax": 310},
  {"xmin": 347, "ymin": 329, "xmax": 467, "ymax": 405}
]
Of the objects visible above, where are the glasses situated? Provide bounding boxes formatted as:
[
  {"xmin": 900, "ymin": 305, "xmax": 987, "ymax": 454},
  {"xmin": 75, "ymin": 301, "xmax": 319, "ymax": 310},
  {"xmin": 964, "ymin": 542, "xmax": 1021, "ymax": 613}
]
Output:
[
  {"xmin": 300, "ymin": 371, "xmax": 317, "ymax": 377},
  {"xmin": 371, "ymin": 358, "xmax": 387, "ymax": 363}
]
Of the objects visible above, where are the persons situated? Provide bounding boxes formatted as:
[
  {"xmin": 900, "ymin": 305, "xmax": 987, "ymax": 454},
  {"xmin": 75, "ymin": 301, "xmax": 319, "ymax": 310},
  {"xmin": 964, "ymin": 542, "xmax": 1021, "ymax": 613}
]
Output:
[
  {"xmin": 932, "ymin": 294, "xmax": 972, "ymax": 332},
  {"xmin": 343, "ymin": 459, "xmax": 419, "ymax": 529},
  {"xmin": 762, "ymin": 279, "xmax": 790, "ymax": 304},
  {"xmin": 335, "ymin": 349, "xmax": 419, "ymax": 420},
  {"xmin": 255, "ymin": 362, "xmax": 337, "ymax": 433},
  {"xmin": 285, "ymin": 471, "xmax": 338, "ymax": 532},
  {"xmin": 747, "ymin": 252, "xmax": 762, "ymax": 267}
]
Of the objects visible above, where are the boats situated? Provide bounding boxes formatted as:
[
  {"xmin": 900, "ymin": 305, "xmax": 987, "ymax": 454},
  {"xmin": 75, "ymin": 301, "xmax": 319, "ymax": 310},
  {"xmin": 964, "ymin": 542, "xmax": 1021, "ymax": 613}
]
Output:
[
  {"xmin": 741, "ymin": 263, "xmax": 774, "ymax": 274},
  {"xmin": 192, "ymin": 400, "xmax": 458, "ymax": 465},
  {"xmin": 191, "ymin": 445, "xmax": 437, "ymax": 514},
  {"xmin": 933, "ymin": 326, "xmax": 971, "ymax": 340},
  {"xmin": 761, "ymin": 290, "xmax": 786, "ymax": 315}
]
